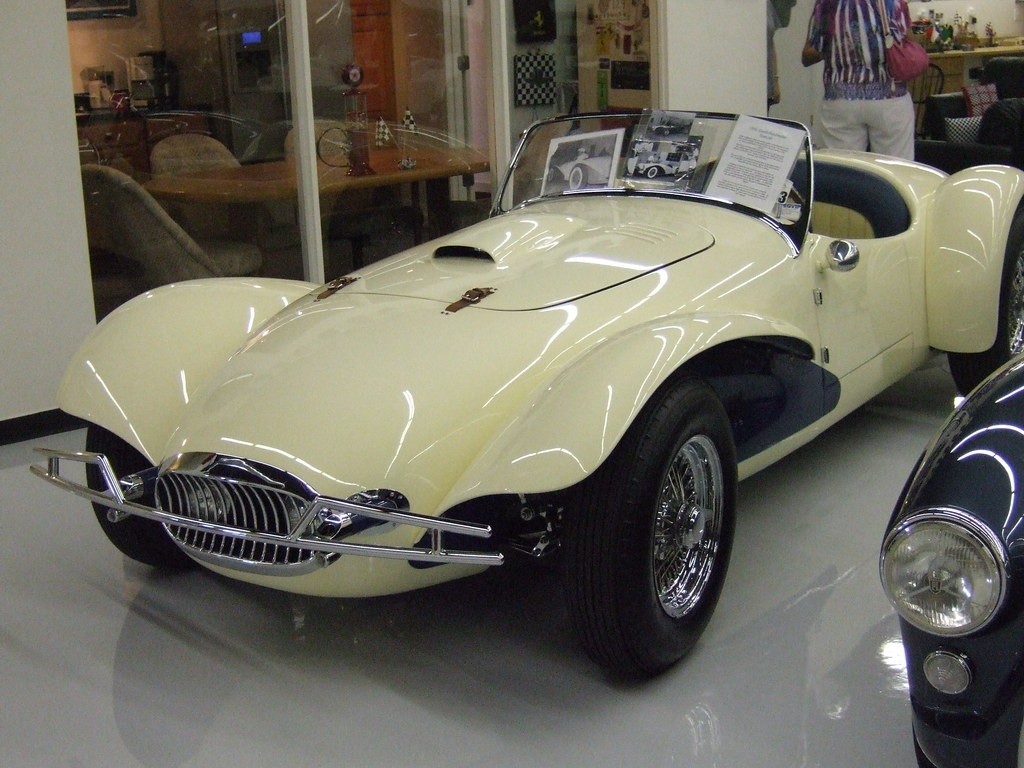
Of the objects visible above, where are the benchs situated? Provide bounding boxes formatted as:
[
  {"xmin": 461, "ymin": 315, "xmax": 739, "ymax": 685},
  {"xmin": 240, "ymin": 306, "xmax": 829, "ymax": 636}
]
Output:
[{"xmin": 684, "ymin": 157, "xmax": 913, "ymax": 237}]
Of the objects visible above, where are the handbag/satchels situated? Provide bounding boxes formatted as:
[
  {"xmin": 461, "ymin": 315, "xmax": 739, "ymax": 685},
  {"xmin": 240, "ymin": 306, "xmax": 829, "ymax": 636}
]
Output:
[{"xmin": 884, "ymin": 34, "xmax": 930, "ymax": 82}]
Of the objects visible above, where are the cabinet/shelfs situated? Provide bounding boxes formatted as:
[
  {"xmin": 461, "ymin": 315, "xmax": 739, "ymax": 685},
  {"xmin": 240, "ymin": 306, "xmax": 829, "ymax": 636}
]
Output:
[{"xmin": 76, "ymin": 103, "xmax": 211, "ymax": 170}]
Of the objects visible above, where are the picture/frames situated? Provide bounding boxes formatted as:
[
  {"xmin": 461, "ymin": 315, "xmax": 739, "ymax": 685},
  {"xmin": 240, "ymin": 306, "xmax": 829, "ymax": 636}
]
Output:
[{"xmin": 66, "ymin": 0, "xmax": 137, "ymax": 20}]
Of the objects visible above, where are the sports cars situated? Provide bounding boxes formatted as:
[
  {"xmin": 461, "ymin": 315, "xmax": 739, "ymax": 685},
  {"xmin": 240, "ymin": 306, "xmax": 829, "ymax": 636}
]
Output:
[{"xmin": 27, "ymin": 110, "xmax": 1023, "ymax": 682}]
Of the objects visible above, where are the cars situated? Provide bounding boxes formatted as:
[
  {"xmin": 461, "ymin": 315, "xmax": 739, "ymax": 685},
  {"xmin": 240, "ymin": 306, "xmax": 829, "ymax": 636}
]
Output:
[{"xmin": 880, "ymin": 351, "xmax": 1023, "ymax": 767}]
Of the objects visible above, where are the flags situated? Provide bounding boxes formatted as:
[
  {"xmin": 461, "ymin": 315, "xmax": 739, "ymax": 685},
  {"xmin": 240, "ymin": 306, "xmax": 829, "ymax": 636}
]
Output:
[
  {"xmin": 375, "ymin": 115, "xmax": 391, "ymax": 149},
  {"xmin": 401, "ymin": 110, "xmax": 419, "ymax": 136}
]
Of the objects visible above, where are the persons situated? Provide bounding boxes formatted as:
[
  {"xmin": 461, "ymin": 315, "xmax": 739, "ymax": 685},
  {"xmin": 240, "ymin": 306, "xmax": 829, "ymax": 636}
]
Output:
[
  {"xmin": 646, "ymin": 153, "xmax": 656, "ymax": 163},
  {"xmin": 801, "ymin": 0, "xmax": 915, "ymax": 162},
  {"xmin": 576, "ymin": 148, "xmax": 589, "ymax": 161}
]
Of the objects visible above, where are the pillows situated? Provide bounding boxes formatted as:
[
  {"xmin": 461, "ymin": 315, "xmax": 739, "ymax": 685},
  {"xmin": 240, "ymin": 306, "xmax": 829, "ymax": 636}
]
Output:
[
  {"xmin": 959, "ymin": 83, "xmax": 999, "ymax": 115},
  {"xmin": 944, "ymin": 116, "xmax": 982, "ymax": 145}
]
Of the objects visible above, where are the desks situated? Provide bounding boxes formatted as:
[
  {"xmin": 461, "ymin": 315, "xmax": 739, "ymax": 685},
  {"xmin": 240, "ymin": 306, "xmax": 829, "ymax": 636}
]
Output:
[{"xmin": 142, "ymin": 147, "xmax": 531, "ymax": 244}]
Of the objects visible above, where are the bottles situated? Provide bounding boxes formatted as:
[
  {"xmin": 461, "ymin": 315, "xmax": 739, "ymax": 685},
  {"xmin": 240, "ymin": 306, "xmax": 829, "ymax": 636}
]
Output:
[{"xmin": 929, "ymin": 7, "xmax": 978, "ymax": 40}]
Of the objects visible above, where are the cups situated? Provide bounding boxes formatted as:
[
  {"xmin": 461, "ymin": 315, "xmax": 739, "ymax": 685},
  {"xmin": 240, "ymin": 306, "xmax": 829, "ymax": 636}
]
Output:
[
  {"xmin": 110, "ymin": 92, "xmax": 129, "ymax": 114},
  {"xmin": 89, "ymin": 79, "xmax": 102, "ymax": 109}
]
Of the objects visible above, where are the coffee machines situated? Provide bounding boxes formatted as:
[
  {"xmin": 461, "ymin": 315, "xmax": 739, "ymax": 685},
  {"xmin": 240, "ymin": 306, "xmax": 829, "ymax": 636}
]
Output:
[{"xmin": 125, "ymin": 55, "xmax": 157, "ymax": 107}]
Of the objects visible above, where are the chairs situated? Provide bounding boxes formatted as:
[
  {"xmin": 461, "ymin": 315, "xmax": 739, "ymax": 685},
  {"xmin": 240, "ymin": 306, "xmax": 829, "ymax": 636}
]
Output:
[
  {"xmin": 81, "ymin": 162, "xmax": 263, "ymax": 293},
  {"xmin": 904, "ymin": 63, "xmax": 945, "ymax": 137},
  {"xmin": 286, "ymin": 118, "xmax": 374, "ymax": 266},
  {"xmin": 151, "ymin": 133, "xmax": 242, "ymax": 238}
]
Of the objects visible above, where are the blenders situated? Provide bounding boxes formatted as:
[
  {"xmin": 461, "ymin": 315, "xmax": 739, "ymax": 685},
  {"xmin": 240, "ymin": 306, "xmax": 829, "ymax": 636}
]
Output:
[{"xmin": 158, "ymin": 62, "xmax": 179, "ymax": 111}]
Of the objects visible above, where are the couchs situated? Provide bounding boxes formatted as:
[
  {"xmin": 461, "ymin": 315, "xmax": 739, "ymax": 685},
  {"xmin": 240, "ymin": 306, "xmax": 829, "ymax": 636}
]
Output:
[{"xmin": 924, "ymin": 56, "xmax": 1024, "ymax": 148}]
